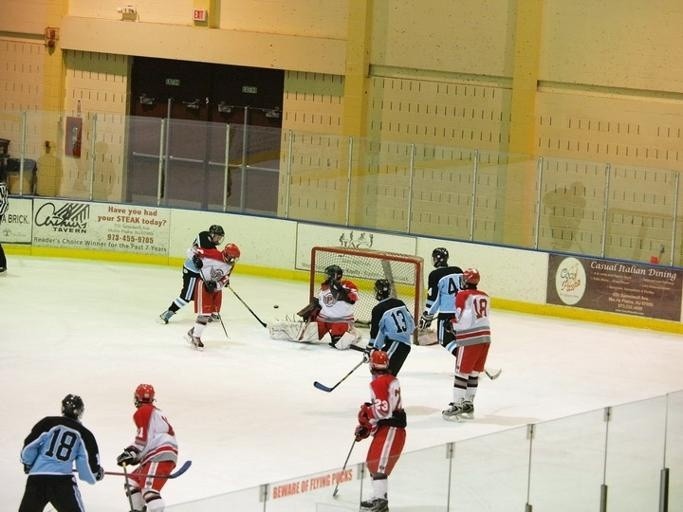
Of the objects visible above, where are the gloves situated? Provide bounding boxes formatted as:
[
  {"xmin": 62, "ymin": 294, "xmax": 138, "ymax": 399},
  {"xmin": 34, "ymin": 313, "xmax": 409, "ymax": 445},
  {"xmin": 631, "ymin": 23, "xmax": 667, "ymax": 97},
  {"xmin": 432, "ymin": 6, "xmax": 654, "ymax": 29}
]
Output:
[
  {"xmin": 205, "ymin": 280, "xmax": 217, "ymax": 293},
  {"xmin": 194, "ymin": 258, "xmax": 203, "ymax": 269},
  {"xmin": 117, "ymin": 445, "xmax": 140, "ymax": 465},
  {"xmin": 354, "ymin": 425, "xmax": 370, "ymax": 441},
  {"xmin": 358, "ymin": 406, "xmax": 375, "ymax": 426},
  {"xmin": 96, "ymin": 467, "xmax": 104, "ymax": 481}
]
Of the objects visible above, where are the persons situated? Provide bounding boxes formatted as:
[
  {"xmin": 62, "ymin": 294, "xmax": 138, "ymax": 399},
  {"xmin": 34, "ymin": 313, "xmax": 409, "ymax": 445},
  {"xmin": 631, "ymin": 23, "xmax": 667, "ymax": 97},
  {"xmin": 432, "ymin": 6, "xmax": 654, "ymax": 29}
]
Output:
[
  {"xmin": 185, "ymin": 243, "xmax": 240, "ymax": 351},
  {"xmin": 159, "ymin": 225, "xmax": 224, "ymax": 324},
  {"xmin": 363, "ymin": 278, "xmax": 416, "ymax": 377},
  {"xmin": 18, "ymin": 394, "xmax": 104, "ymax": 511},
  {"xmin": 417, "ymin": 248, "xmax": 464, "ymax": 358},
  {"xmin": 355, "ymin": 351, "xmax": 407, "ymax": 512},
  {"xmin": 0, "ymin": 177, "xmax": 8, "ymax": 272},
  {"xmin": 116, "ymin": 384, "xmax": 178, "ymax": 511},
  {"xmin": 442, "ymin": 267, "xmax": 491, "ymax": 425},
  {"xmin": 303, "ymin": 265, "xmax": 359, "ymax": 349}
]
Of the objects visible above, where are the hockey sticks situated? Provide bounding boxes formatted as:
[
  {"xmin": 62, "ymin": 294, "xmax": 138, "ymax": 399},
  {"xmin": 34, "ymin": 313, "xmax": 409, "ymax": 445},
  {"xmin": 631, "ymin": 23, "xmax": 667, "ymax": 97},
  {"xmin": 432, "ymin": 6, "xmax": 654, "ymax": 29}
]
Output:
[
  {"xmin": 313, "ymin": 357, "xmax": 365, "ymax": 392},
  {"xmin": 226, "ymin": 284, "xmax": 272, "ymax": 329},
  {"xmin": 445, "ymin": 324, "xmax": 501, "ymax": 380},
  {"xmin": 72, "ymin": 461, "xmax": 192, "ymax": 478},
  {"xmin": 332, "ymin": 436, "xmax": 355, "ymax": 497},
  {"xmin": 198, "ymin": 266, "xmax": 240, "ymax": 342}
]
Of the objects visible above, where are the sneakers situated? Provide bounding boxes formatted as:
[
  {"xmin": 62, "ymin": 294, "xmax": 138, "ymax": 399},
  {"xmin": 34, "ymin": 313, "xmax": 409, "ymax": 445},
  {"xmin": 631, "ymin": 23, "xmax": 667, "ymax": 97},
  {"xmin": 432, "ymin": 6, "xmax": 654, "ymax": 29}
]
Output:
[
  {"xmin": 463, "ymin": 401, "xmax": 474, "ymax": 413},
  {"xmin": 159, "ymin": 308, "xmax": 174, "ymax": 326},
  {"xmin": 362, "ymin": 497, "xmax": 376, "ymax": 508},
  {"xmin": 187, "ymin": 328, "xmax": 204, "ymax": 351},
  {"xmin": 442, "ymin": 405, "xmax": 463, "ymax": 416},
  {"xmin": 371, "ymin": 500, "xmax": 388, "ymax": 511},
  {"xmin": 210, "ymin": 314, "xmax": 220, "ymax": 321}
]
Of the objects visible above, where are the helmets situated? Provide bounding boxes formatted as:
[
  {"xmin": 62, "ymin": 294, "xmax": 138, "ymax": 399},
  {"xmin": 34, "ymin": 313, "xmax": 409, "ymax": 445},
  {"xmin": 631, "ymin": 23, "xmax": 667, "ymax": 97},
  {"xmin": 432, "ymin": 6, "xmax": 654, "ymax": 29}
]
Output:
[
  {"xmin": 374, "ymin": 279, "xmax": 390, "ymax": 299},
  {"xmin": 135, "ymin": 384, "xmax": 155, "ymax": 403},
  {"xmin": 223, "ymin": 244, "xmax": 240, "ymax": 263},
  {"xmin": 432, "ymin": 247, "xmax": 448, "ymax": 268},
  {"xmin": 209, "ymin": 225, "xmax": 225, "ymax": 245},
  {"xmin": 369, "ymin": 348, "xmax": 388, "ymax": 370},
  {"xmin": 61, "ymin": 394, "xmax": 84, "ymax": 417},
  {"xmin": 324, "ymin": 266, "xmax": 342, "ymax": 282},
  {"xmin": 463, "ymin": 268, "xmax": 480, "ymax": 286}
]
